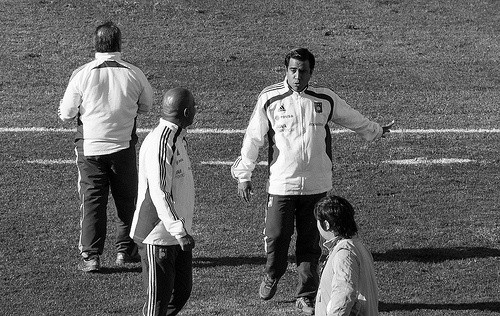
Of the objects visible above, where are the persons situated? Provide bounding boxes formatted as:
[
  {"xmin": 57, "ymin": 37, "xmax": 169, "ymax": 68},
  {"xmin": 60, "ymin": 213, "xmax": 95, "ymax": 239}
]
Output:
[
  {"xmin": 129, "ymin": 87, "xmax": 198, "ymax": 316},
  {"xmin": 227, "ymin": 47, "xmax": 394, "ymax": 316},
  {"xmin": 57, "ymin": 22, "xmax": 155, "ymax": 274},
  {"xmin": 311, "ymin": 195, "xmax": 381, "ymax": 316}
]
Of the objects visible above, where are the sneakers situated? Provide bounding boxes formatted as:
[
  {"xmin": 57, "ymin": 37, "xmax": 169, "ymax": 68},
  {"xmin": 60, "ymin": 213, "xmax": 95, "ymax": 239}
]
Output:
[
  {"xmin": 77, "ymin": 258, "xmax": 100, "ymax": 272},
  {"xmin": 295, "ymin": 297, "xmax": 315, "ymax": 316},
  {"xmin": 259, "ymin": 270, "xmax": 280, "ymax": 300},
  {"xmin": 115, "ymin": 252, "xmax": 141, "ymax": 264}
]
